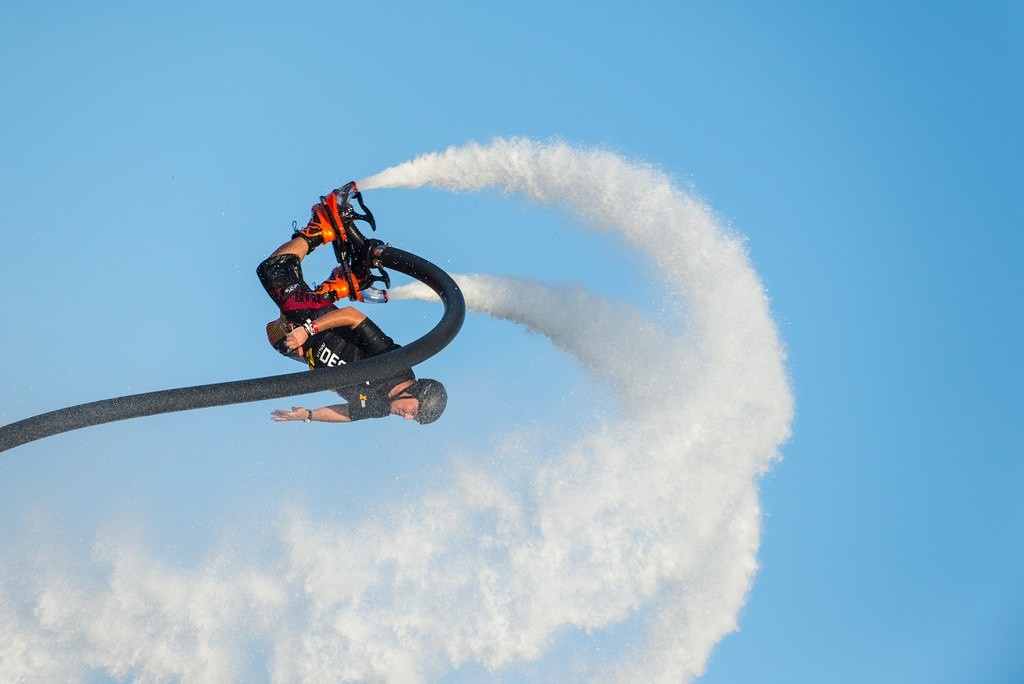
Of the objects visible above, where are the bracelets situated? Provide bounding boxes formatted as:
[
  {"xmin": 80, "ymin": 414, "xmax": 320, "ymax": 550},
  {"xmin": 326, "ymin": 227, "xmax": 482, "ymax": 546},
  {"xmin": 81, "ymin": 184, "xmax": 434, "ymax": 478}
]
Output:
[{"xmin": 303, "ymin": 318, "xmax": 318, "ymax": 335}]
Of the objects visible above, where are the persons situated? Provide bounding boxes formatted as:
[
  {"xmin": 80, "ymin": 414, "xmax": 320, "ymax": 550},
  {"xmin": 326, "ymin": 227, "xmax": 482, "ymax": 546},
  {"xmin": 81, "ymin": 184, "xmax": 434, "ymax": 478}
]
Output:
[{"xmin": 256, "ymin": 204, "xmax": 447, "ymax": 425}]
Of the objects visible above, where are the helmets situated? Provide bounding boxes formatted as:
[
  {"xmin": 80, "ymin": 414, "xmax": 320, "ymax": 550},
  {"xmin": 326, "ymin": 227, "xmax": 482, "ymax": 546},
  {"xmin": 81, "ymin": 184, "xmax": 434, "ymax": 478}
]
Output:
[{"xmin": 407, "ymin": 378, "xmax": 448, "ymax": 425}]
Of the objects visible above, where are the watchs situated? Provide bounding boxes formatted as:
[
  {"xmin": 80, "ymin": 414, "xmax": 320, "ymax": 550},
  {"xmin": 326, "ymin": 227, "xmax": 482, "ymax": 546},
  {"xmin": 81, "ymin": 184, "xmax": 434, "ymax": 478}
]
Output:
[{"xmin": 303, "ymin": 409, "xmax": 313, "ymax": 423}]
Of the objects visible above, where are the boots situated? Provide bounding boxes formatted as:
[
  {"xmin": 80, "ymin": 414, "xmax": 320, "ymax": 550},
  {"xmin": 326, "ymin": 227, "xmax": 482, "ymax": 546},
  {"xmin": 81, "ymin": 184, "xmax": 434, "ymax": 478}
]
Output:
[
  {"xmin": 315, "ymin": 264, "xmax": 364, "ymax": 301},
  {"xmin": 291, "ymin": 203, "xmax": 335, "ymax": 255}
]
